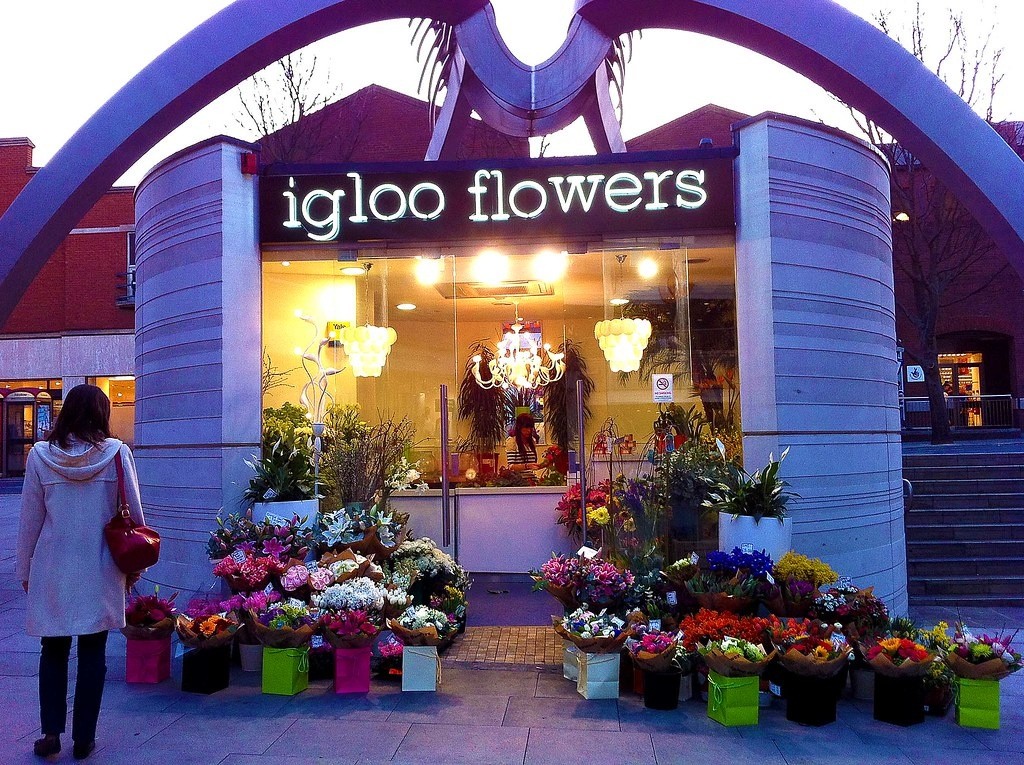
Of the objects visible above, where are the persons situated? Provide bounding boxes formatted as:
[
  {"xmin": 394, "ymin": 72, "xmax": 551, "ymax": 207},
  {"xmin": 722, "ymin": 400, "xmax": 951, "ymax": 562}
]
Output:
[
  {"xmin": 505, "ymin": 413, "xmax": 541, "ymax": 482},
  {"xmin": 941, "ymin": 380, "xmax": 968, "ymax": 425},
  {"xmin": 898, "ymin": 380, "xmax": 905, "ymax": 435},
  {"xmin": 16, "ymin": 385, "xmax": 147, "ymax": 759}
]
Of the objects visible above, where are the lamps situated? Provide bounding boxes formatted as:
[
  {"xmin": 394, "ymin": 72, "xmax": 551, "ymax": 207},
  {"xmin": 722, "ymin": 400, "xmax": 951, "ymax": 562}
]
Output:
[
  {"xmin": 472, "ymin": 302, "xmax": 565, "ymax": 389},
  {"xmin": 339, "ymin": 263, "xmax": 397, "ymax": 376},
  {"xmin": 595, "ymin": 252, "xmax": 652, "ymax": 374}
]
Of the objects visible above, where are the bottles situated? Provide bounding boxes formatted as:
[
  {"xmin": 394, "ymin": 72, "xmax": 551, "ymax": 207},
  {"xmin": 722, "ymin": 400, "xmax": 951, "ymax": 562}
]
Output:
[{"xmin": 620, "ymin": 433, "xmax": 637, "ymax": 454}]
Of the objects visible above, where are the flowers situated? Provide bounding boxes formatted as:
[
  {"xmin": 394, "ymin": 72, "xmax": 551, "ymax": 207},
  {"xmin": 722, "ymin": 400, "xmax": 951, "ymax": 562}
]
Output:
[{"xmin": 123, "ymin": 402, "xmax": 1024, "ymax": 666}]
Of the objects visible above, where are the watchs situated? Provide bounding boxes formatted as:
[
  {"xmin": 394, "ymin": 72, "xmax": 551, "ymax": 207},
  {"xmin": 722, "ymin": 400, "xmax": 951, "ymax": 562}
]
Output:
[{"xmin": 525, "ymin": 463, "xmax": 530, "ymax": 470}]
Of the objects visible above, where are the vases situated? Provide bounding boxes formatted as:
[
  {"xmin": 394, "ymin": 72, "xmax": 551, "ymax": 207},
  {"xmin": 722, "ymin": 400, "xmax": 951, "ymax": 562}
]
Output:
[
  {"xmin": 238, "ymin": 643, "xmax": 266, "ymax": 671},
  {"xmin": 719, "ymin": 510, "xmax": 793, "ymax": 570},
  {"xmin": 252, "ymin": 498, "xmax": 318, "ymax": 538},
  {"xmin": 927, "ymin": 685, "xmax": 954, "ymax": 718},
  {"xmin": 222, "ymin": 574, "xmax": 276, "ymax": 597},
  {"xmin": 850, "ymin": 666, "xmax": 874, "ymax": 697}
]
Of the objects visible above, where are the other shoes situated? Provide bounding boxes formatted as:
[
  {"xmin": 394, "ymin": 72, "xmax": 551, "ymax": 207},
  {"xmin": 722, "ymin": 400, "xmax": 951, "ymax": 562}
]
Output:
[
  {"xmin": 34, "ymin": 737, "xmax": 61, "ymax": 756},
  {"xmin": 73, "ymin": 739, "xmax": 95, "ymax": 758}
]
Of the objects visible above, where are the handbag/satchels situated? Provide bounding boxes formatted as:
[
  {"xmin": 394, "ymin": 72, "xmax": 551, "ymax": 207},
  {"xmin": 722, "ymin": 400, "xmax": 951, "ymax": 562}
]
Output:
[{"xmin": 104, "ymin": 451, "xmax": 160, "ymax": 574}]
